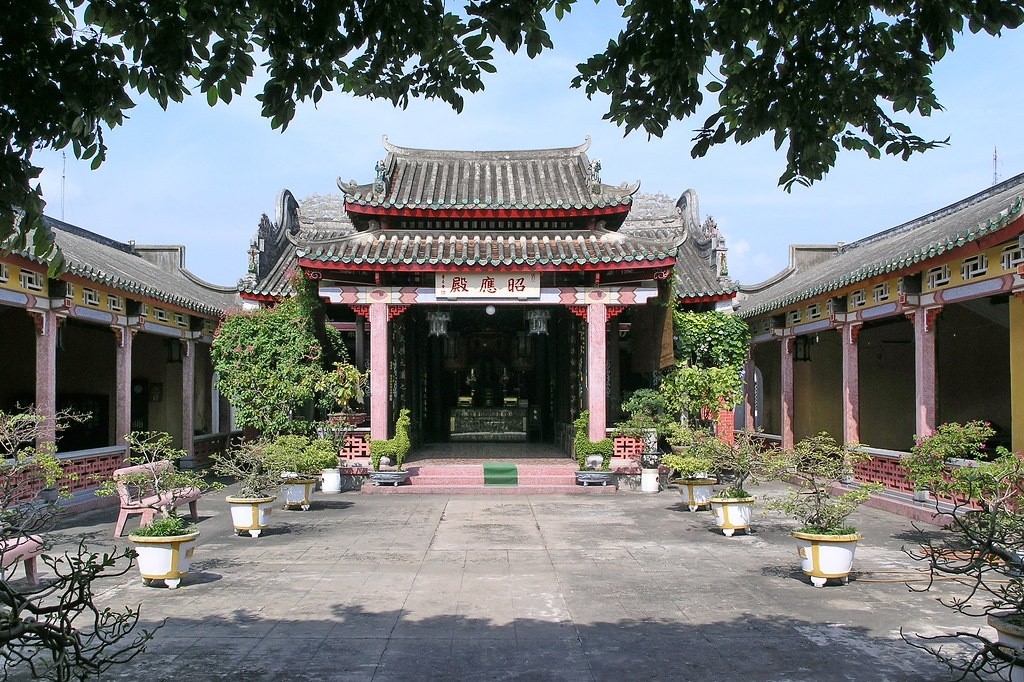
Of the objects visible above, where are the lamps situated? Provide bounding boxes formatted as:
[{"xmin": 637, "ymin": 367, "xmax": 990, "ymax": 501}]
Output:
[{"xmin": 485, "ymin": 305, "xmax": 495, "ymax": 314}]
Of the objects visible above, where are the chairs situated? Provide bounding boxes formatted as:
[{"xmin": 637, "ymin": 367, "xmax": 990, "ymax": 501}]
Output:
[{"xmin": 528, "ymin": 405, "xmax": 543, "ymax": 442}]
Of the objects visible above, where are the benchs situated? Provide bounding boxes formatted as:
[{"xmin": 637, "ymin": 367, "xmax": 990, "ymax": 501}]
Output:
[
  {"xmin": 0, "ymin": 534, "xmax": 44, "ymax": 587},
  {"xmin": 113, "ymin": 460, "xmax": 202, "ymax": 538}
]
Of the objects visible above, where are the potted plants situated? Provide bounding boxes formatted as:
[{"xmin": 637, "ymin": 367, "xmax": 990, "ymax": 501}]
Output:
[
  {"xmin": 314, "ymin": 361, "xmax": 370, "ymax": 426},
  {"xmin": 665, "ymin": 421, "xmax": 696, "ymax": 456},
  {"xmin": 572, "ymin": 410, "xmax": 618, "ymax": 486},
  {"xmin": 899, "ymin": 461, "xmax": 1023, "ymax": 682},
  {"xmin": 610, "ymin": 411, "xmax": 675, "ymax": 469},
  {"xmin": 363, "ymin": 408, "xmax": 412, "ymax": 487},
  {"xmin": 298, "ymin": 420, "xmax": 352, "ymax": 495},
  {"xmin": 208, "ymin": 436, "xmax": 293, "ymax": 538},
  {"xmin": 260, "ymin": 435, "xmax": 339, "ymax": 511},
  {"xmin": 86, "ymin": 431, "xmax": 228, "ymax": 588},
  {"xmin": 661, "ymin": 444, "xmax": 716, "ymax": 512},
  {"xmin": 746, "ymin": 431, "xmax": 886, "ymax": 588},
  {"xmin": 700, "ymin": 425, "xmax": 779, "ymax": 536}
]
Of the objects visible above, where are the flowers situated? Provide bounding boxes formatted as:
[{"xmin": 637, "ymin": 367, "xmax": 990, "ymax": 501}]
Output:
[{"xmin": 897, "ymin": 420, "xmax": 1023, "ymax": 536}]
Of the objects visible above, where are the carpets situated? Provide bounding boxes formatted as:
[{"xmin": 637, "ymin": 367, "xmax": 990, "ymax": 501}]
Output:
[{"xmin": 482, "ymin": 462, "xmax": 518, "ymax": 486}]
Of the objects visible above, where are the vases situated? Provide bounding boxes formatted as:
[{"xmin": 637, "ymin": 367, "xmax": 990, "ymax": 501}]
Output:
[{"xmin": 964, "ymin": 510, "xmax": 1002, "ymax": 548}]
